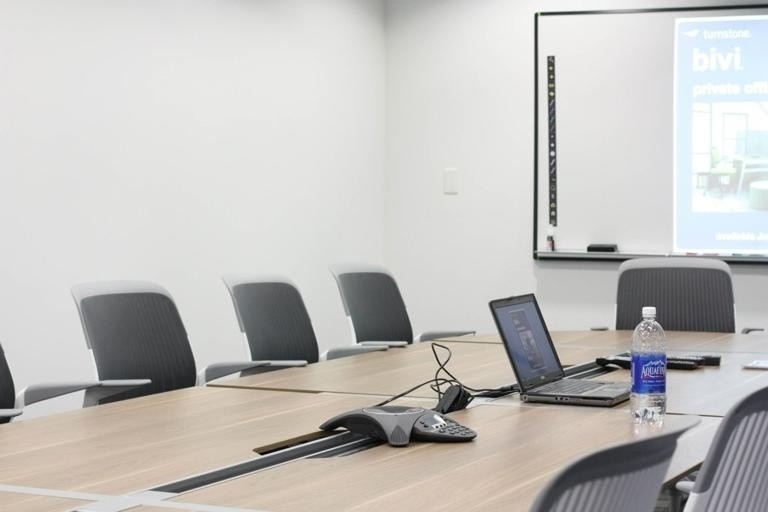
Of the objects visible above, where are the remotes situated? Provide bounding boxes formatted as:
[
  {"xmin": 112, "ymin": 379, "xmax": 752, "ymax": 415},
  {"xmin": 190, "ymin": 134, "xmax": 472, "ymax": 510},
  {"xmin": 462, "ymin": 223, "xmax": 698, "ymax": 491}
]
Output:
[
  {"xmin": 667, "ymin": 352, "xmax": 721, "ymax": 366},
  {"xmin": 666, "ymin": 355, "xmax": 705, "ymax": 366},
  {"xmin": 596, "ymin": 354, "xmax": 699, "ymax": 370}
]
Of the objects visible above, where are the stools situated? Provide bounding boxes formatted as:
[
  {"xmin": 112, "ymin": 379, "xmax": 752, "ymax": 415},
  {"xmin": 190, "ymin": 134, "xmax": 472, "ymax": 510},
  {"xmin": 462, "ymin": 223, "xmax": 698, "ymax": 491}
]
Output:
[
  {"xmin": 748, "ymin": 180, "xmax": 768, "ymax": 212},
  {"xmin": 691, "ymin": 167, "xmax": 738, "ymax": 201}
]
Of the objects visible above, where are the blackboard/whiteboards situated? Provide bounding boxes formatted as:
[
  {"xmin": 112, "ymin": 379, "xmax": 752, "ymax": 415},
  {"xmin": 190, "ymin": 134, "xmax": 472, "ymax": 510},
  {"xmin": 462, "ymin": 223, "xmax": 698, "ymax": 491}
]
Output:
[{"xmin": 531, "ymin": 4, "xmax": 768, "ymax": 264}]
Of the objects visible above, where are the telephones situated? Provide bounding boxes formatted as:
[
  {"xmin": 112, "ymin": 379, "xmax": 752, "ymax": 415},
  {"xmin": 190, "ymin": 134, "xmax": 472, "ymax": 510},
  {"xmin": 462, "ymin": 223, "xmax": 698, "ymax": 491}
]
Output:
[{"xmin": 319, "ymin": 405, "xmax": 477, "ymax": 446}]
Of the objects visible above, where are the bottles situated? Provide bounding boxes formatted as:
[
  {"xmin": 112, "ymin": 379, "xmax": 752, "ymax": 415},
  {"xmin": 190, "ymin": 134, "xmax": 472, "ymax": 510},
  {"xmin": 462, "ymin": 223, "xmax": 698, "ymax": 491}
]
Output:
[{"xmin": 628, "ymin": 305, "xmax": 669, "ymax": 429}]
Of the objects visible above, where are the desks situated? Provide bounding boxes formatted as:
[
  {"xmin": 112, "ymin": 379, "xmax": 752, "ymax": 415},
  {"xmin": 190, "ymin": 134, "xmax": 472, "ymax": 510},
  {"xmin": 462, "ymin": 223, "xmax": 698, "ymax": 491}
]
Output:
[
  {"xmin": 0, "ymin": 329, "xmax": 768, "ymax": 512},
  {"xmin": 726, "ymin": 155, "xmax": 768, "ymax": 199}
]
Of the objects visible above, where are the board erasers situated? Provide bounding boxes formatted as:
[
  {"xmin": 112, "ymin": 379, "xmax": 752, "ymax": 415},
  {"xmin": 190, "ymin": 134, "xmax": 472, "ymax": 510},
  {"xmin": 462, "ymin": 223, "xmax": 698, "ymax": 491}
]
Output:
[{"xmin": 587, "ymin": 244, "xmax": 617, "ymax": 252}]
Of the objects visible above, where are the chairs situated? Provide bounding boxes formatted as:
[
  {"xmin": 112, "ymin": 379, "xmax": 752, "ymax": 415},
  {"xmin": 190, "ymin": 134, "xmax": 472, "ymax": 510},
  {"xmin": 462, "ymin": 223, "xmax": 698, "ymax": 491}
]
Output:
[
  {"xmin": 589, "ymin": 256, "xmax": 765, "ymax": 335},
  {"xmin": 220, "ymin": 270, "xmax": 389, "ymax": 379},
  {"xmin": 0, "ymin": 343, "xmax": 104, "ymax": 425},
  {"xmin": 328, "ymin": 261, "xmax": 477, "ymax": 347},
  {"xmin": 667, "ymin": 385, "xmax": 768, "ymax": 512},
  {"xmin": 69, "ymin": 279, "xmax": 271, "ymax": 409},
  {"xmin": 529, "ymin": 413, "xmax": 703, "ymax": 512}
]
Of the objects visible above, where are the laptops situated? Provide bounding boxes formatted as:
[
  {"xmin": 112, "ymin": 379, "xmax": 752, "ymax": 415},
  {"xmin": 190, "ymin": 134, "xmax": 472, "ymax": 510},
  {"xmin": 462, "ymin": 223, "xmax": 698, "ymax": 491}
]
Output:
[{"xmin": 488, "ymin": 292, "xmax": 631, "ymax": 407}]
